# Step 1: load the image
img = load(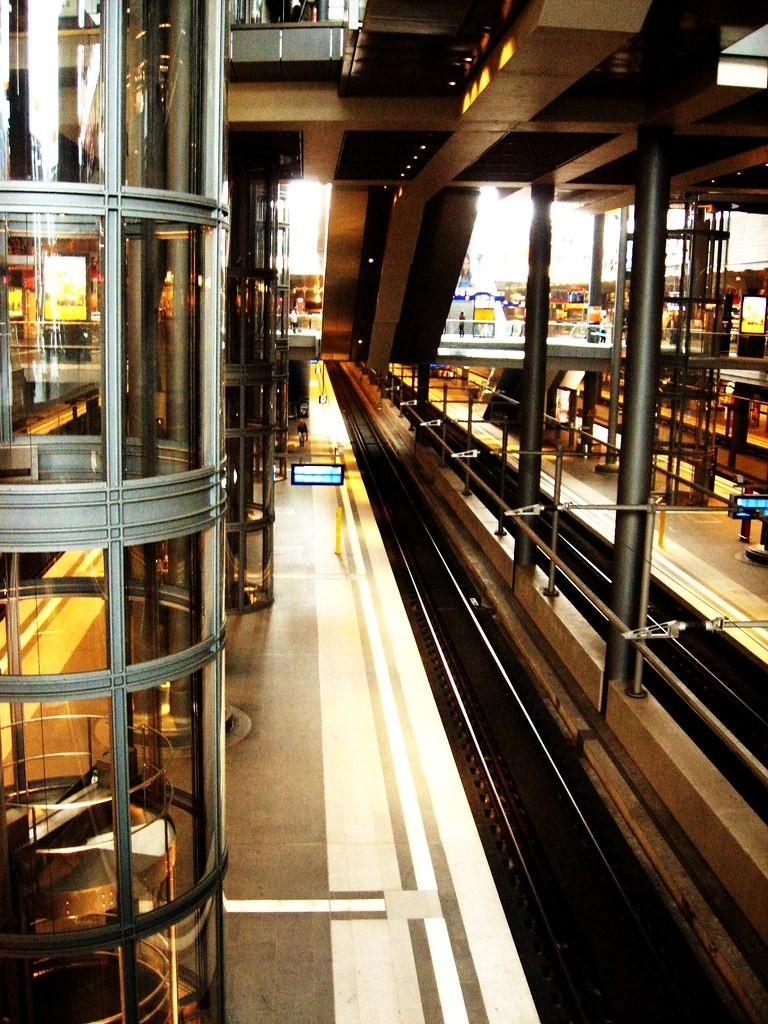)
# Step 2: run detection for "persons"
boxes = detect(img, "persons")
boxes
[570,292,582,303]
[666,314,676,344]
[599,314,607,343]
[457,254,474,287]
[297,419,308,447]
[458,311,465,338]
[291,309,298,333]
